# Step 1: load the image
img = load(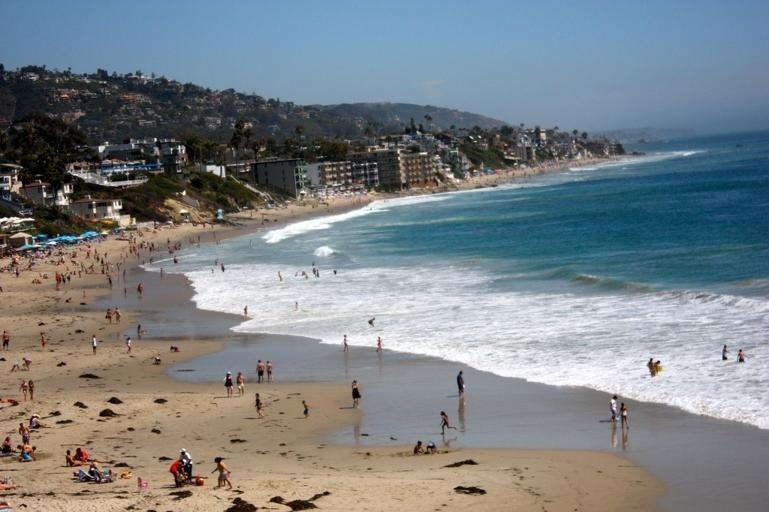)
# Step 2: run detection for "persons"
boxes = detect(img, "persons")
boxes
[438,410,457,435]
[722,344,729,360]
[646,358,655,377]
[609,395,618,420]
[442,434,457,448]
[611,423,619,447]
[412,439,424,454]
[458,392,465,432]
[621,425,629,450]
[737,349,747,362]
[654,360,661,374]
[617,402,629,427]
[425,440,438,454]
[457,371,464,392]
[1,211,387,489]
